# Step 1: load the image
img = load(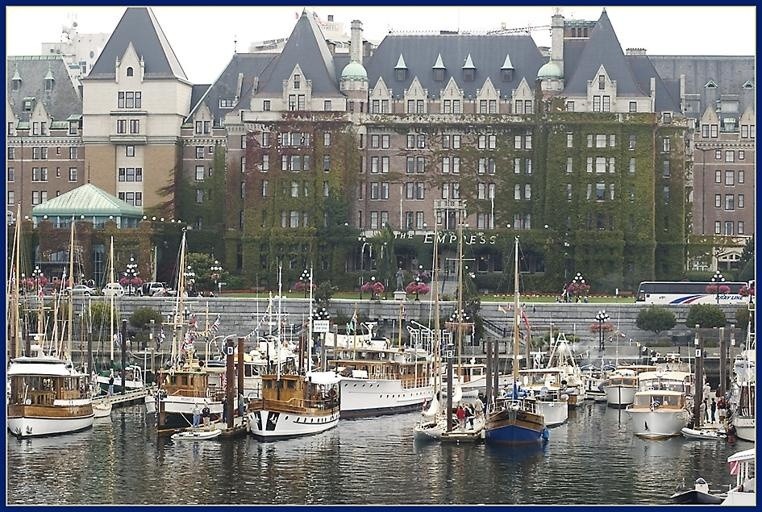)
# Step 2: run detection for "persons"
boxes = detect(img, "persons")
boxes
[395,267,406,293]
[238,392,244,418]
[558,293,590,303]
[191,402,202,429]
[222,395,228,425]
[700,382,730,426]
[455,391,490,422]
[156,333,161,353]
[201,402,211,428]
[107,374,114,397]
